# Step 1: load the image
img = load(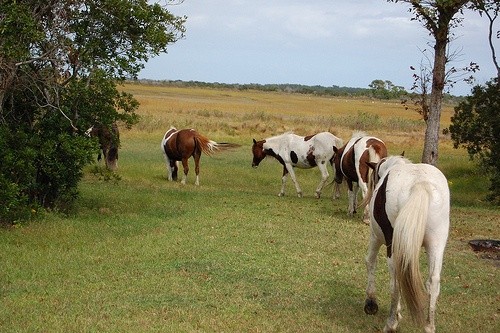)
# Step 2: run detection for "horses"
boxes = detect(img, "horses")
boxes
[330,137,388,225]
[251,132,345,200]
[362,148,450,333]
[160,127,244,187]
[83,122,121,174]
